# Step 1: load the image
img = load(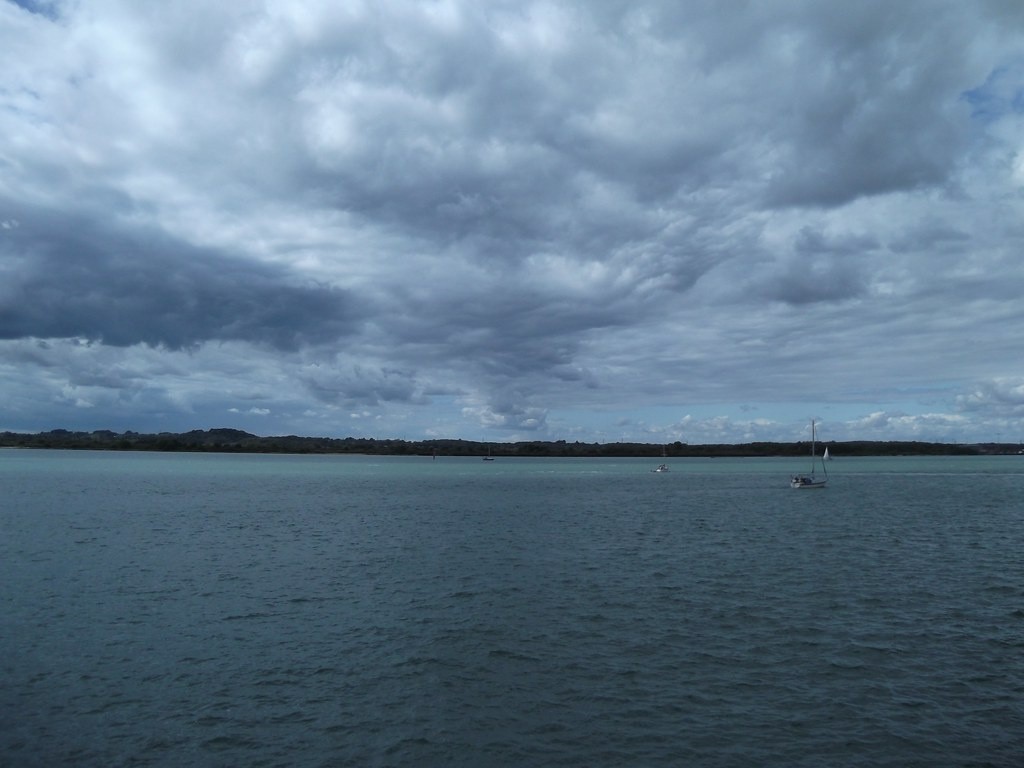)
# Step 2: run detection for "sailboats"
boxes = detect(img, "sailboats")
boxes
[651,443,670,473]
[483,441,494,461]
[791,420,829,489]
[822,447,832,461]
